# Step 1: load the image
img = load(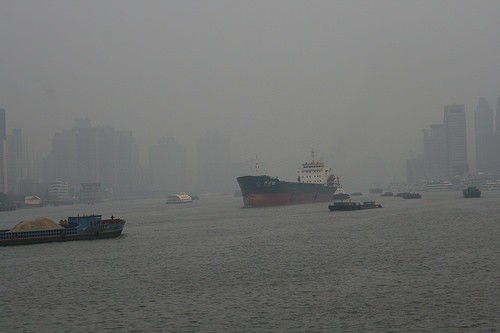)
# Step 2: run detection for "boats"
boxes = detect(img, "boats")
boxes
[166,192,193,203]
[332,185,351,200]
[235,150,341,207]
[463,186,481,198]
[328,199,384,211]
[0,214,127,247]
[351,192,363,197]
[380,192,423,200]
